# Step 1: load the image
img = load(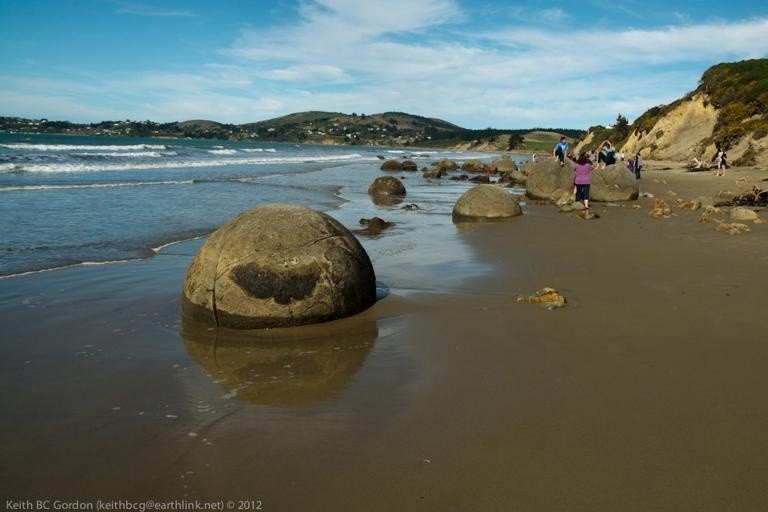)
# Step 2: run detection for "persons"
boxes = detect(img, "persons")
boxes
[626,158,633,173]
[552,136,568,167]
[714,146,728,177]
[531,152,536,162]
[572,151,592,209]
[566,149,625,165]
[594,140,618,170]
[571,168,585,202]
[633,151,642,179]
